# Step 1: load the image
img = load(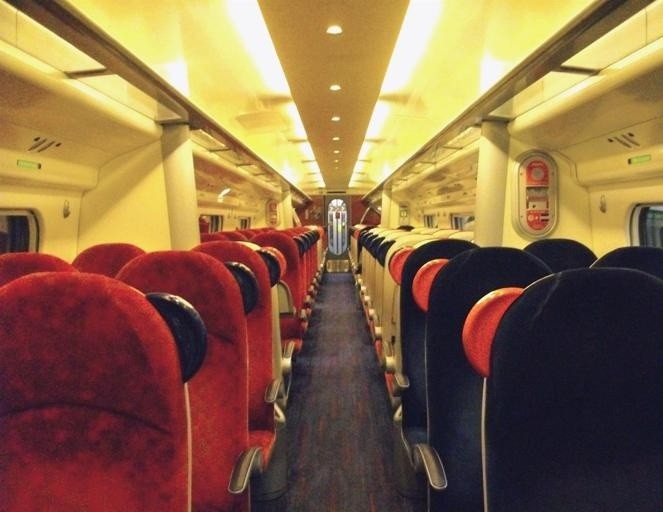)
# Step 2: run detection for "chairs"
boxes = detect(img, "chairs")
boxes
[347,225,439,448]
[461,266,662,512]
[0,252,79,285]
[74,244,146,276]
[1,272,206,512]
[410,247,554,512]
[387,239,491,490]
[200,226,276,240]
[525,239,593,277]
[254,226,331,332]
[412,225,472,239]
[590,245,661,274]
[117,252,259,512]
[193,240,283,449]
